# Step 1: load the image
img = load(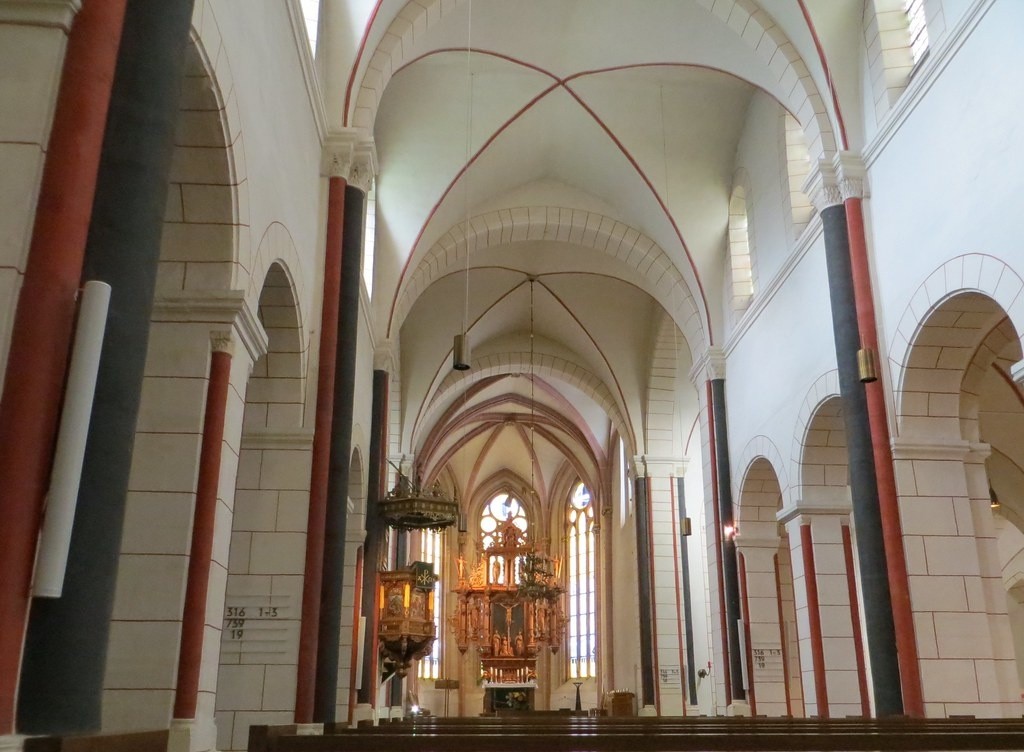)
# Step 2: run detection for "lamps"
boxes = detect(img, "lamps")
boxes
[858,346,877,384]
[453,334,471,370]
[681,518,691,535]
[988,479,1002,507]
